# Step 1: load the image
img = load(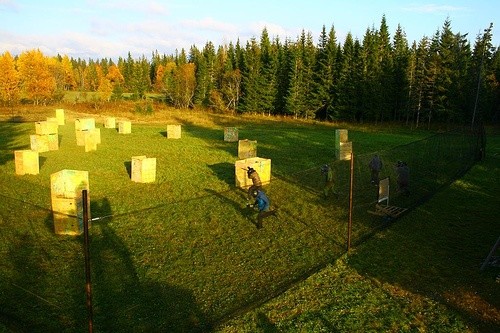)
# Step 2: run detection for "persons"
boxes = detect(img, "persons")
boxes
[247,168,262,200]
[369,154,383,183]
[324,166,339,199]
[395,162,409,194]
[252,190,276,229]
